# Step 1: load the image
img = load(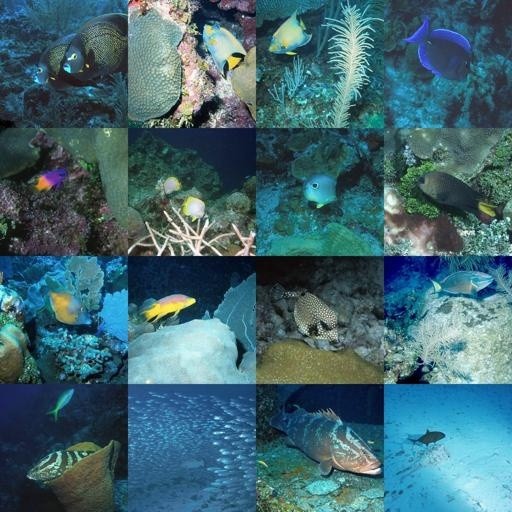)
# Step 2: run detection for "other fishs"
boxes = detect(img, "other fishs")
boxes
[429,270,495,299]
[33,167,67,194]
[32,13,128,88]
[269,408,382,477]
[417,170,508,225]
[26,450,96,482]
[202,23,247,80]
[47,288,94,327]
[402,16,473,83]
[293,287,339,344]
[164,175,207,223]
[138,293,197,326]
[268,9,314,57]
[407,429,446,447]
[180,459,206,470]
[303,174,338,209]
[45,388,75,423]
